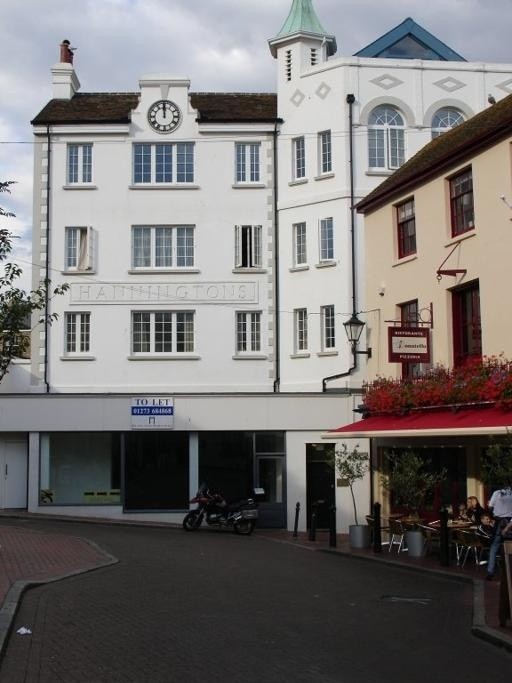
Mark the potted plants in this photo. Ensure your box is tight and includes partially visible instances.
[324,442,371,548]
[376,448,449,557]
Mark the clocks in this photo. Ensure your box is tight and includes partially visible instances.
[147,98,183,134]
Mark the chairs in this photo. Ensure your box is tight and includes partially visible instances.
[387,517,491,568]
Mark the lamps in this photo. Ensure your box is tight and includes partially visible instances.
[343,311,372,358]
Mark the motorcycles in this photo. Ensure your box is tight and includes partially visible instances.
[182,480,260,535]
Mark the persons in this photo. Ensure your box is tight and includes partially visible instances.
[443,476,512,580]
